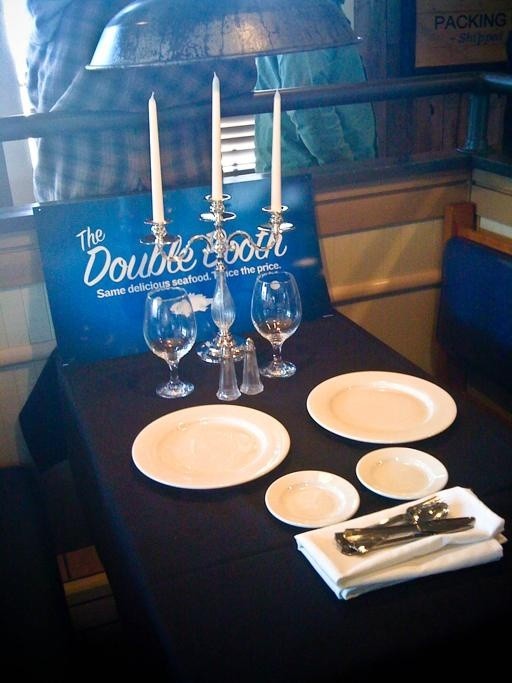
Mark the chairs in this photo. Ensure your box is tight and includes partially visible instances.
[423,193,511,436]
[18,174,335,542]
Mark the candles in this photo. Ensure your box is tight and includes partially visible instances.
[147,88,165,222]
[209,68,223,200]
[271,86,282,210]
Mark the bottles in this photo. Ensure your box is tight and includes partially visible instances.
[242,340,267,395]
[216,344,242,404]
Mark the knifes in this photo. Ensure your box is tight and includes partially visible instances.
[335,495,477,555]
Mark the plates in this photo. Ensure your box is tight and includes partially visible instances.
[132,404,292,489]
[305,370,458,445]
[356,445,447,505]
[264,469,360,528]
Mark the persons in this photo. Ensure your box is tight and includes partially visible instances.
[23,0,258,203]
[254,0,378,173]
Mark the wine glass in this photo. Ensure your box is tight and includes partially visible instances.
[252,271,304,378]
[144,285,197,397]
